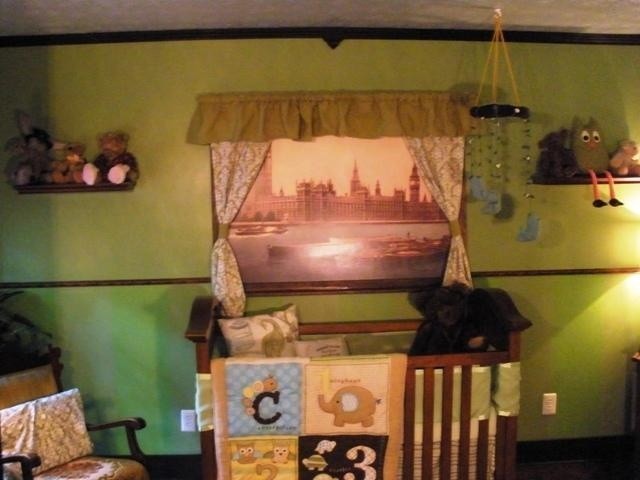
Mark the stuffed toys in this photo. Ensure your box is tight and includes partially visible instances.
[536,116,640,207]
[1,110,139,190]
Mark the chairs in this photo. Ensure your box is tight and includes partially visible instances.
[0,363,147,480]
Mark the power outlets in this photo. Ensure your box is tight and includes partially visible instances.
[542,393,557,416]
[181,410,196,433]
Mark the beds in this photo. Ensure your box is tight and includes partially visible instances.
[184,289,526,478]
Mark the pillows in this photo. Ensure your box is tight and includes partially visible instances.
[295,338,352,360]
[2,388,93,479]
[218,305,297,357]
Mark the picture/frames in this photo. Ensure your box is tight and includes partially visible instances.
[194,89,466,291]
